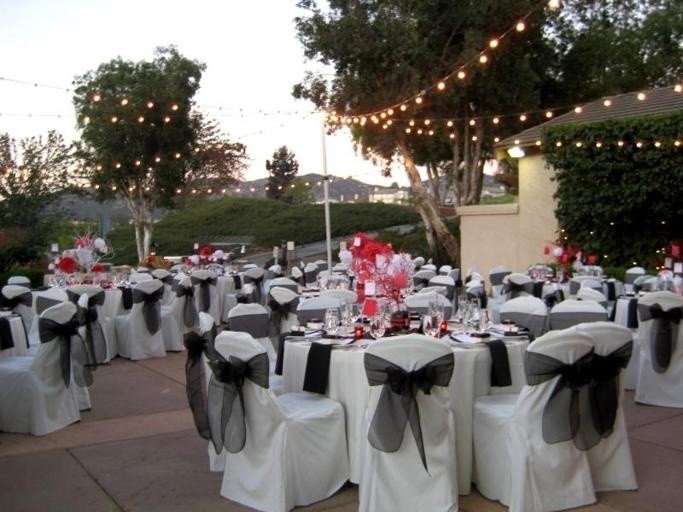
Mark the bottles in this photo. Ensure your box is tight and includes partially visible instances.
[353,324,363,337]
[408,316,421,328]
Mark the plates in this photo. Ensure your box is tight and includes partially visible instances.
[449,329,502,344]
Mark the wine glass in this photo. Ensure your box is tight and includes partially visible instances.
[345,299,364,338]
[457,286,468,321]
[428,301,445,338]
[339,298,348,333]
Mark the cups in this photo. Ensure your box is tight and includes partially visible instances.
[422,314,439,338]
[290,325,305,340]
[368,316,387,339]
[324,307,339,335]
[306,317,322,330]
[409,310,420,316]
[500,320,515,331]
[470,299,481,320]
